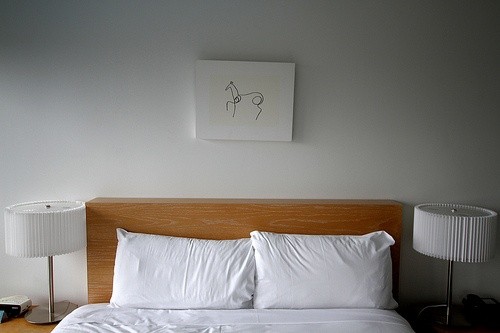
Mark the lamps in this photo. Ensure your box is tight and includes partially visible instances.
[413,202,498,326]
[5,200,86,324]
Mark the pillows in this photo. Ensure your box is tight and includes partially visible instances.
[250,230,398,309]
[107,228,256,309]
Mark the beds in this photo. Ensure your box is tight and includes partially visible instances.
[50,197,416,333]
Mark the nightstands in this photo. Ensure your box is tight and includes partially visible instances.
[401,301,500,333]
[0,306,59,333]
